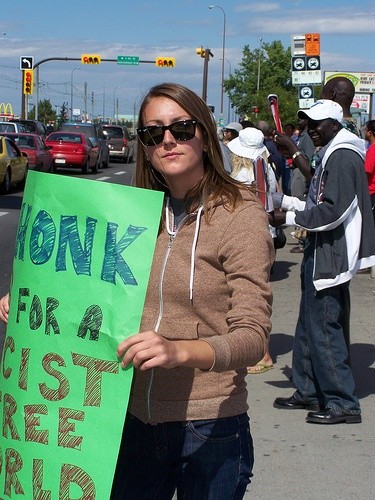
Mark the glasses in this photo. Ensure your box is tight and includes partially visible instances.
[135,119,201,147]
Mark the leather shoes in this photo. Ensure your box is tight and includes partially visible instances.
[272,395,321,412]
[306,408,362,424]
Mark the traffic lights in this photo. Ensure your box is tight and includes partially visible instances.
[24,69,33,95]
[196,45,205,58]
[156,57,176,67]
[252,106,259,113]
[81,54,101,65]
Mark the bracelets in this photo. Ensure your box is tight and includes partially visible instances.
[292,150,303,159]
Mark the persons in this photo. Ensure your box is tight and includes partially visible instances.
[268,98,375,425]
[1,82,276,500]
[221,78,374,251]
[226,127,278,374]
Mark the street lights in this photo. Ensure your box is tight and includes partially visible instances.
[208,4,226,127]
[71,67,81,122]
[219,58,232,124]
[114,86,121,118]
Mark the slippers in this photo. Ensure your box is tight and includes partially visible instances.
[247,363,275,374]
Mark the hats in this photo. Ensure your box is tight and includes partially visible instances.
[224,122,244,134]
[297,98,344,126]
[227,127,270,161]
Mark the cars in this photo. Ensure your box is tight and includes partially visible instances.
[0,136,29,194]
[43,131,100,174]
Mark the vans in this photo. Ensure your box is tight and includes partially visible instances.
[58,121,111,169]
[0,119,55,145]
[102,124,136,163]
